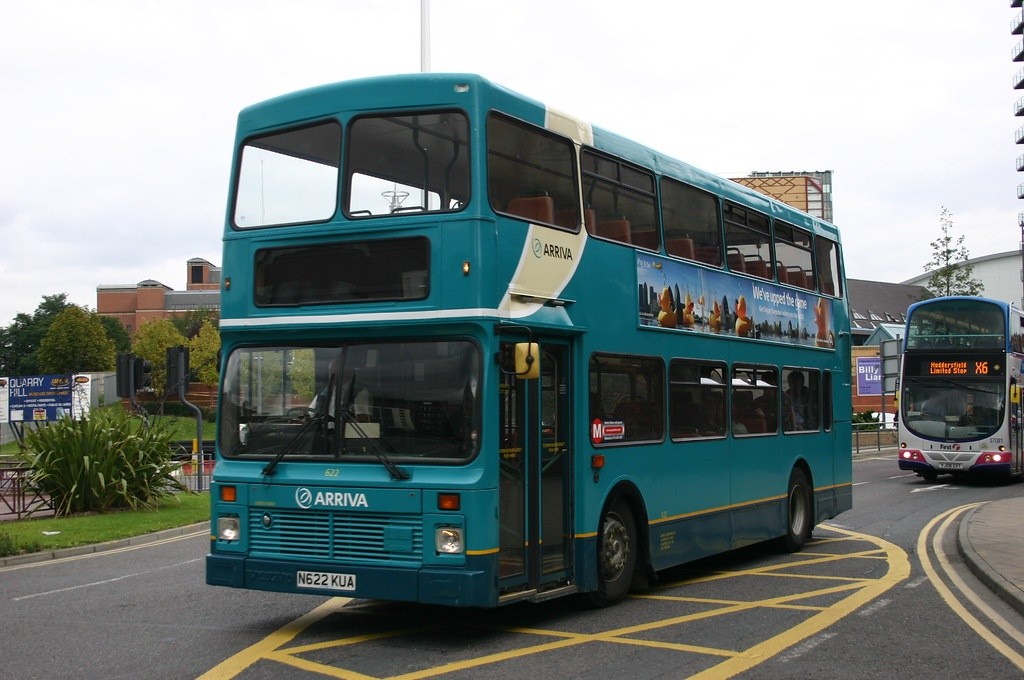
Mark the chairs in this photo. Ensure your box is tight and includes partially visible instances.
[554,203,596,235]
[694,246,719,266]
[736,416,767,434]
[611,396,645,425]
[804,270,826,293]
[631,231,659,251]
[727,248,746,273]
[786,266,807,289]
[744,255,768,279]
[666,231,695,260]
[596,214,632,243]
[765,261,788,284]
[506,189,554,225]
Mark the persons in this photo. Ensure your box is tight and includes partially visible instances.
[308,358,375,424]
[920,393,947,417]
[697,369,816,437]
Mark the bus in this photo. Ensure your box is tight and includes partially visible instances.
[203,73,853,611]
[897,296,1024,484]
